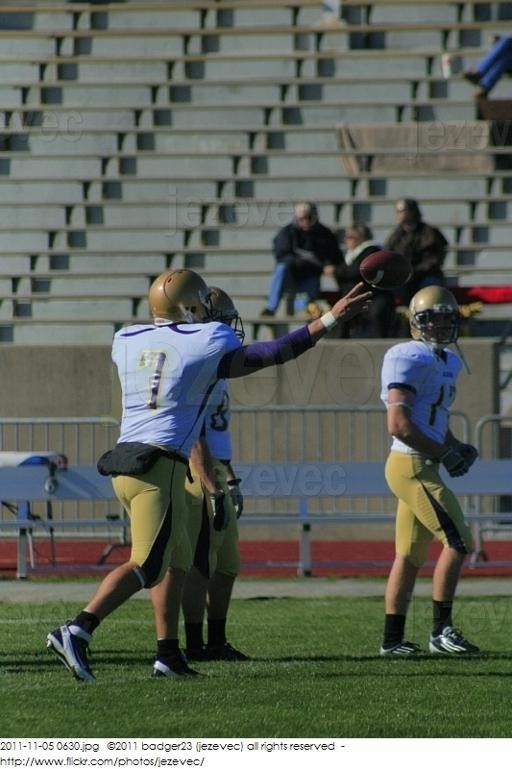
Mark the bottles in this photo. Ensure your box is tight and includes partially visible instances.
[294,293,308,318]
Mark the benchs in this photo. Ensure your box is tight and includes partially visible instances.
[0,0,512,342]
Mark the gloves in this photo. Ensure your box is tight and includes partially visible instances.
[227,475,243,520]
[437,439,477,478]
[206,489,229,531]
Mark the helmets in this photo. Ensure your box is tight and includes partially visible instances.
[149,269,245,345]
[409,285,459,344]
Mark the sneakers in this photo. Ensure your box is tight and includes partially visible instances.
[153,651,203,678]
[429,625,479,653]
[381,641,427,655]
[185,642,250,661]
[44,621,96,680]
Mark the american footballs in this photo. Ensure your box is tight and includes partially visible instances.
[359,250,413,291]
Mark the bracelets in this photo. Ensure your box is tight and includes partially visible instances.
[320,311,337,333]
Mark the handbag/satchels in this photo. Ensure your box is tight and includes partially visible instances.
[97,441,162,473]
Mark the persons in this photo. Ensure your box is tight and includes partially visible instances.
[319,222,396,339]
[180,283,245,663]
[381,285,480,656]
[462,35,512,98]
[382,197,449,293]
[260,200,346,320]
[45,267,374,684]
